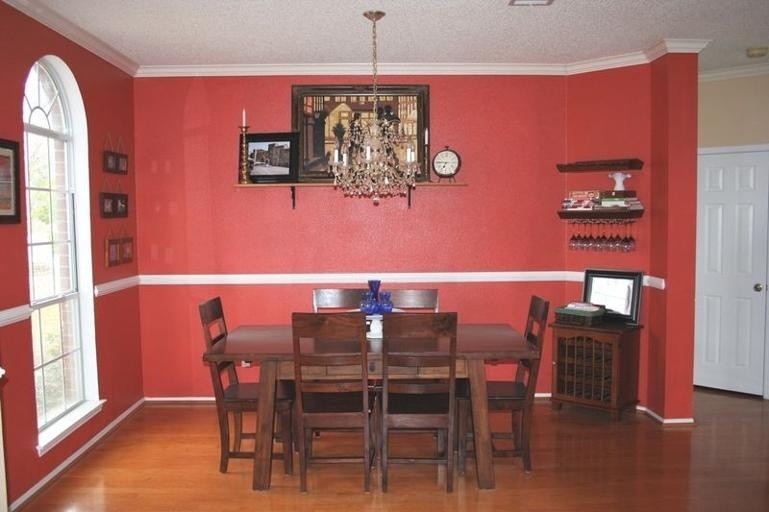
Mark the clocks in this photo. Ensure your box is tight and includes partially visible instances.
[432,146,462,183]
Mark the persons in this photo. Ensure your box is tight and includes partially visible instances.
[383,105,402,129]
[376,107,384,128]
[584,191,599,204]
[119,199,127,213]
[347,112,364,171]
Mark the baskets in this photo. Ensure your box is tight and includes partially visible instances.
[555,305,606,326]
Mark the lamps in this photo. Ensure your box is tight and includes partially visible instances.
[332,10,429,206]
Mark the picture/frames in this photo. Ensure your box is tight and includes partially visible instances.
[100,192,127,217]
[103,151,128,175]
[105,236,134,266]
[291,84,430,183]
[0,139,20,224]
[240,133,298,183]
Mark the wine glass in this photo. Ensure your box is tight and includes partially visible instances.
[569,220,638,251]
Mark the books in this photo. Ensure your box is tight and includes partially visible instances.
[599,190,645,207]
[592,207,645,210]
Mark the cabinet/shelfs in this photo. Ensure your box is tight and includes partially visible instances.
[557,158,644,220]
[548,321,644,421]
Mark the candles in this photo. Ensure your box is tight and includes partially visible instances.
[424,126,429,145]
[242,108,246,126]
